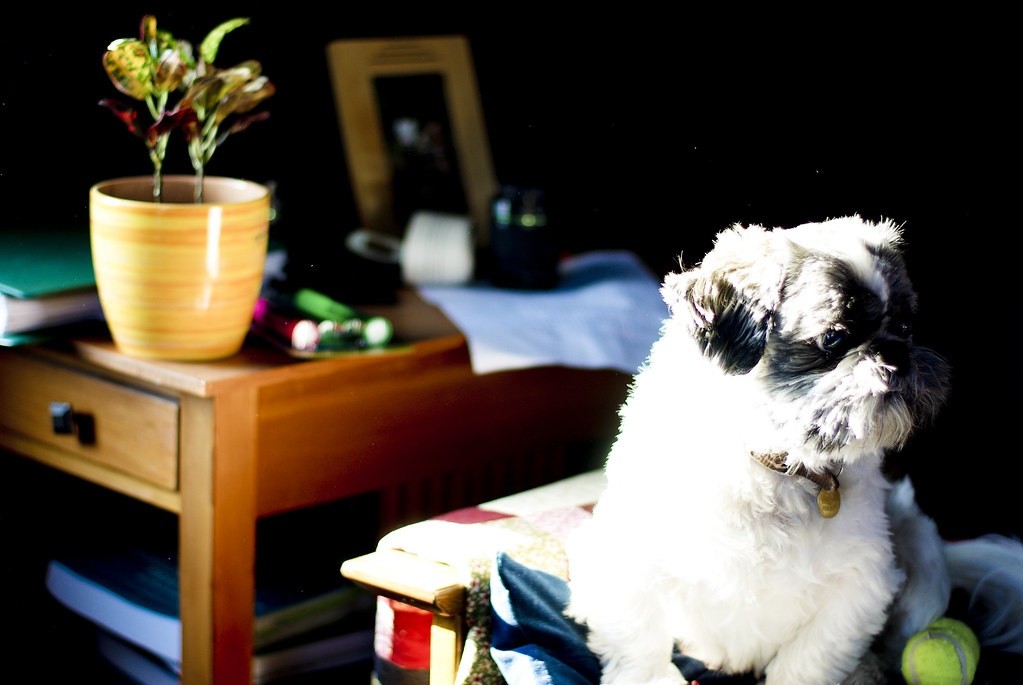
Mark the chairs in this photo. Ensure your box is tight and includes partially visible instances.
[336,467,1023,685]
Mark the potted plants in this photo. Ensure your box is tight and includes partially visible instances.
[89,12,278,365]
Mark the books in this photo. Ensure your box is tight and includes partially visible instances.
[0,230,104,347]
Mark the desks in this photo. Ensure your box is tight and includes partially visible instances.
[0,322,559,685]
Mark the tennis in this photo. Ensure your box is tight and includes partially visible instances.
[901,618,980,685]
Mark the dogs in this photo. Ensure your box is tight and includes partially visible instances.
[560,213,950,685]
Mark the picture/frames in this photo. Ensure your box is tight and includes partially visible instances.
[329,40,498,228]
